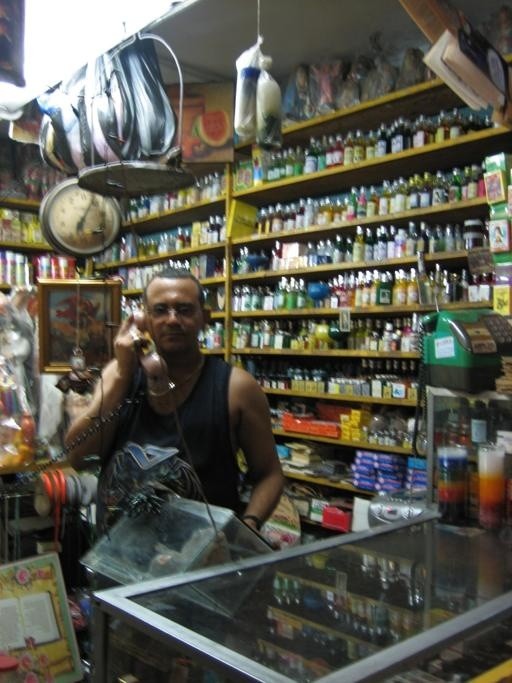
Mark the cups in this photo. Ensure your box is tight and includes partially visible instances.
[434,441,508,533]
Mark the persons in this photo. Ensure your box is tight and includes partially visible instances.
[64,266,285,537]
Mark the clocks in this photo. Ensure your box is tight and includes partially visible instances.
[38,176,126,258]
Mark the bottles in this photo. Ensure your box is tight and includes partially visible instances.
[93,154,225,351]
[230,106,509,399]
[257,554,471,683]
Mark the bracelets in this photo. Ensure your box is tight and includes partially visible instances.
[241,514,264,530]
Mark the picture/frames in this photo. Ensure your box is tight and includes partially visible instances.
[32,276,123,378]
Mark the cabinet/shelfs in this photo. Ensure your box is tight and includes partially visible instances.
[223,53,512,504]
[2,482,68,562]
[260,536,460,681]
[82,512,510,683]
[0,195,87,301]
[119,161,228,359]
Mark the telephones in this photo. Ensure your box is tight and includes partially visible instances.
[124,311,154,357]
[418,305,510,392]
